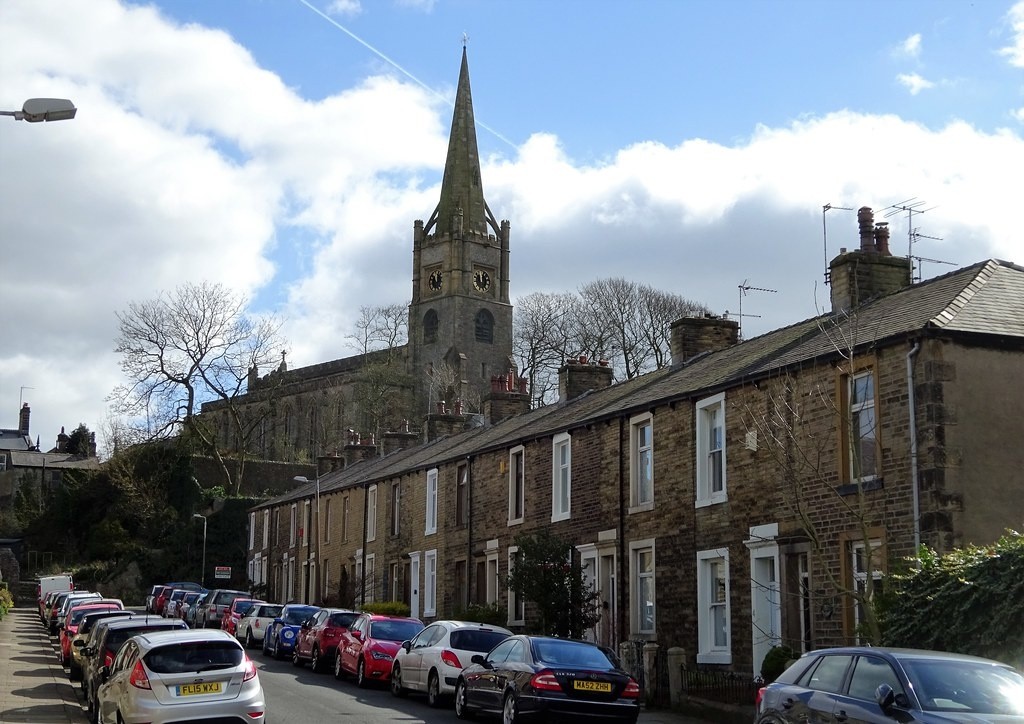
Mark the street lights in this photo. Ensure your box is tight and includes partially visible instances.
[194,514,206,590]
[294,476,322,608]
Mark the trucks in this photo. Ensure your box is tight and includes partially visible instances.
[36,571,73,600]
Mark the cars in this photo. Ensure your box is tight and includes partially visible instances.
[334,612,425,689]
[146,582,284,650]
[754,647,1024,724]
[454,635,641,724]
[39,591,267,724]
[390,621,516,708]
[292,608,362,673]
[263,603,321,661]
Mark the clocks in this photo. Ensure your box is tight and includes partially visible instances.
[428,269,444,293]
[473,270,491,293]
